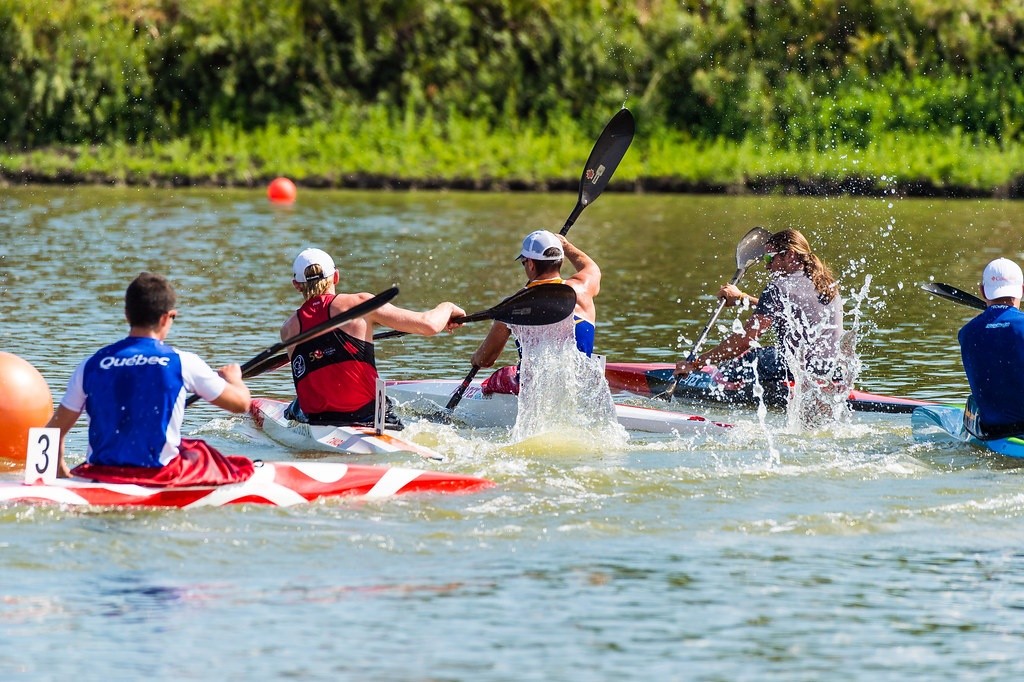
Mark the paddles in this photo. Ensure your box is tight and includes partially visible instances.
[237,282,580,379]
[180,286,406,413]
[437,107,641,427]
[917,275,991,311]
[650,227,778,405]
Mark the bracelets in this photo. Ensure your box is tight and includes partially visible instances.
[738,293,747,306]
[692,357,703,371]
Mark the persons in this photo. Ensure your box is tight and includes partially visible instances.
[44,272,252,478]
[471,229,601,396]
[957,256,1024,440]
[279,248,467,431]
[673,228,843,383]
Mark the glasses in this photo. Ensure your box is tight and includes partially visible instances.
[169,314,175,320]
[521,257,528,265]
[763,250,789,264]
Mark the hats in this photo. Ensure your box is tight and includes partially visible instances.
[515,229,564,261]
[292,247,336,283]
[982,256,1024,300]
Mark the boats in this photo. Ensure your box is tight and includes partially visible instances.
[605,360,935,439]
[382,376,735,452]
[910,403,1024,480]
[1,457,495,508]
[249,395,474,460]
